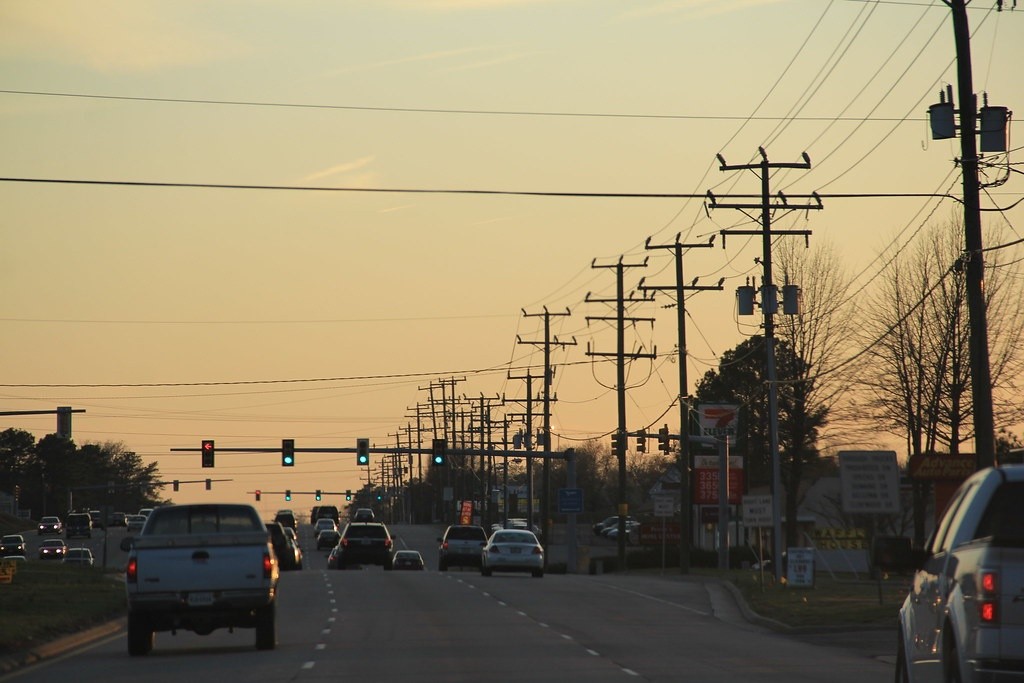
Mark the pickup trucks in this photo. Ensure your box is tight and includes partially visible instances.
[120,503,279,655]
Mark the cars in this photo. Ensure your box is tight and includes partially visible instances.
[0,535,27,560]
[479,529,544,577]
[354,508,374,521]
[38,539,67,560]
[63,548,95,565]
[314,518,341,569]
[89,509,154,532]
[265,509,303,570]
[601,521,641,541]
[491,519,542,539]
[392,549,424,570]
[37,516,62,535]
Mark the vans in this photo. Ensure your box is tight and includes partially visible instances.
[65,513,93,539]
[309,506,341,525]
[897,464,1024,683]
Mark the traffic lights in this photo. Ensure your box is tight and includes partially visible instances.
[376,490,382,501]
[658,424,669,450]
[286,490,291,501]
[202,440,214,467]
[206,479,211,490]
[433,438,445,466]
[282,439,294,466]
[637,429,646,453]
[256,490,261,501]
[316,490,321,501]
[611,433,619,459]
[346,490,351,501]
[357,439,369,465]
[174,480,178,491]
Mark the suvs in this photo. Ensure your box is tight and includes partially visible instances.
[335,522,396,570]
[436,524,488,571]
[592,515,633,536]
